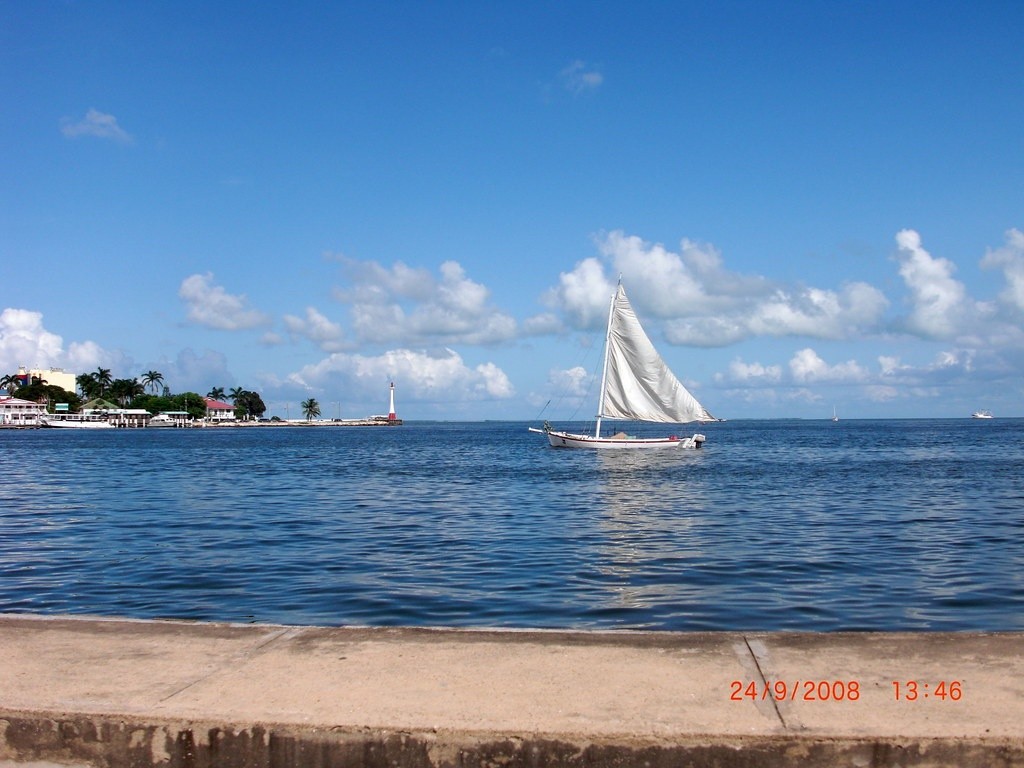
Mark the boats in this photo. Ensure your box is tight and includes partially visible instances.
[40,418,116,428]
[147,414,175,427]
[971,406,995,419]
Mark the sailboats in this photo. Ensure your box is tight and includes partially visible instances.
[832,406,839,422]
[527,271,727,450]
